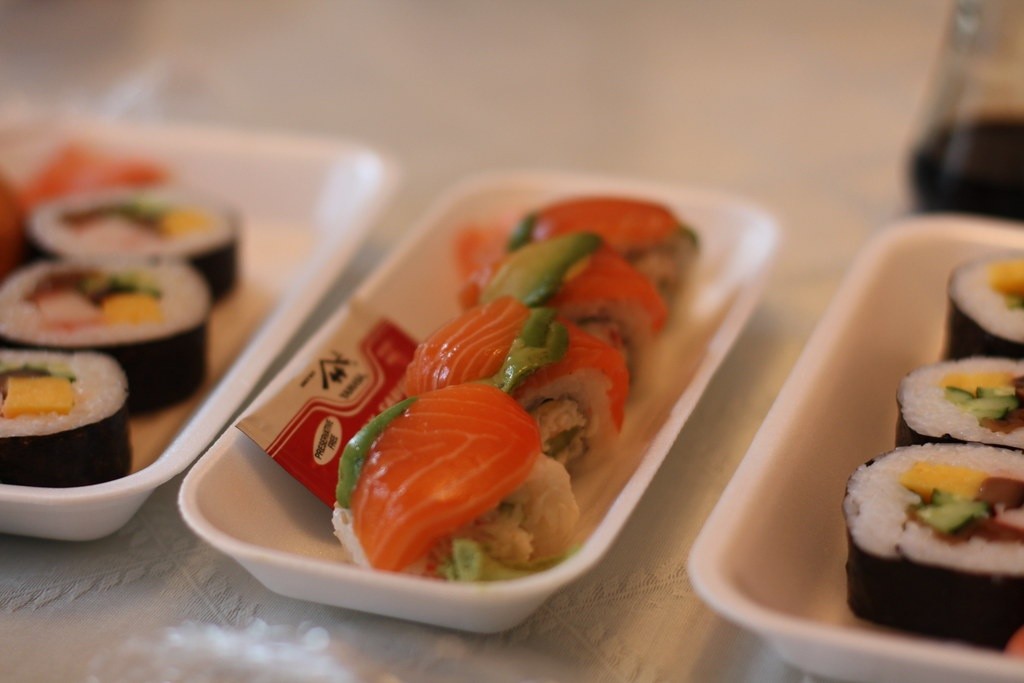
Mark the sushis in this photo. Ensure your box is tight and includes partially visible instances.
[328,198,701,577]
[843,250,1023,654]
[0,182,237,488]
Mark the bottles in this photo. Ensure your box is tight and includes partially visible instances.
[902,0,1024,223]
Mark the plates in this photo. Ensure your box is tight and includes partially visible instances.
[685,214,1024,683]
[176,170,781,633]
[0,97,397,544]
